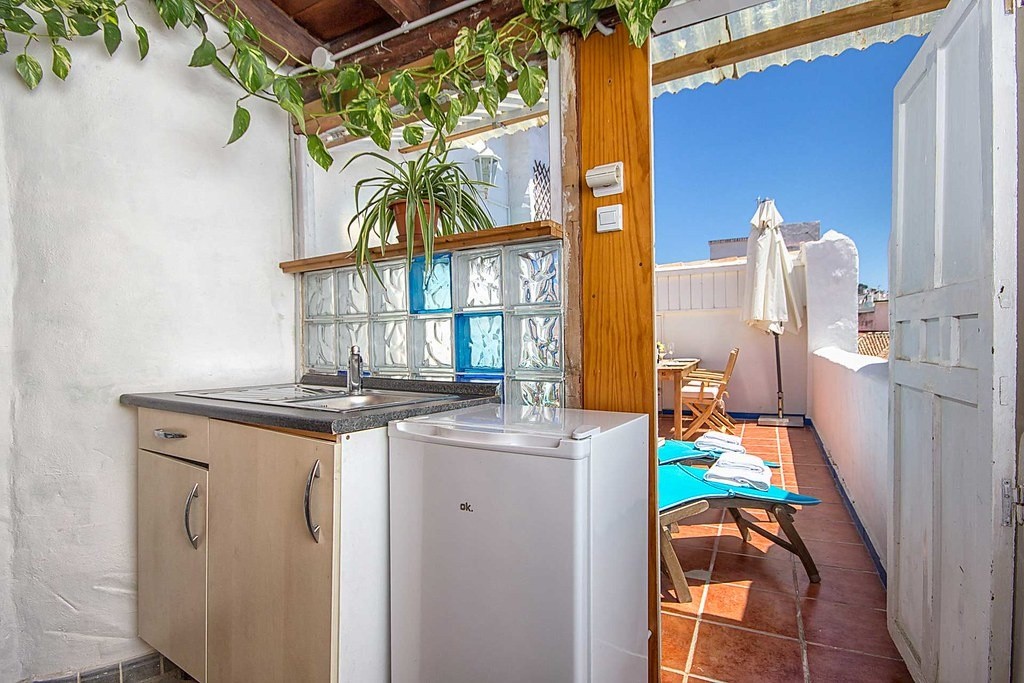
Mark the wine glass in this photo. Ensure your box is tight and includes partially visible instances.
[668,342,675,362]
[658,350,667,364]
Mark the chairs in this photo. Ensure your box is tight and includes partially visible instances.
[657,441,823,604]
[682,347,739,441]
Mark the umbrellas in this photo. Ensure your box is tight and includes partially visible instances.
[739,198,803,418]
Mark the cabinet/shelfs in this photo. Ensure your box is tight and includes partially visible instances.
[121,407,502,682]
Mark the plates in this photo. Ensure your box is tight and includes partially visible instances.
[663,362,687,366]
[673,359,697,362]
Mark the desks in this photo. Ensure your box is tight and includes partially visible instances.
[657,358,700,441]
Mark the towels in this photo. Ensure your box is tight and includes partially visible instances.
[694,430,746,454]
[702,452,771,492]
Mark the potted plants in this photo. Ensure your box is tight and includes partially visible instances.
[339,127,500,293]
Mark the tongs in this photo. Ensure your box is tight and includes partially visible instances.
[294,385,345,396]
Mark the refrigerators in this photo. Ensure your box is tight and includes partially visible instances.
[388,403,649,683]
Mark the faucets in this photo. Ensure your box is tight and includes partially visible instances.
[346,345,364,395]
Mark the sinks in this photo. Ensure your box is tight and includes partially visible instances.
[286,391,460,415]
